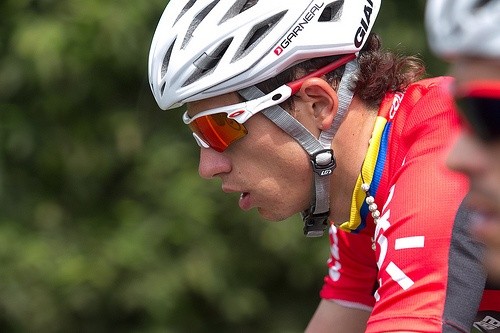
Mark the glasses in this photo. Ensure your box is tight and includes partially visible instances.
[183,52,360,153]
[444,81,500,143]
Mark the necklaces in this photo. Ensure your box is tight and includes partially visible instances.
[360,131,381,250]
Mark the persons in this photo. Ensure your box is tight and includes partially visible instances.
[147,0,475,333]
[424,0,500,333]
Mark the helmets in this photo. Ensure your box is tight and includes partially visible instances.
[149,0,381,111]
[425,0,500,60]
